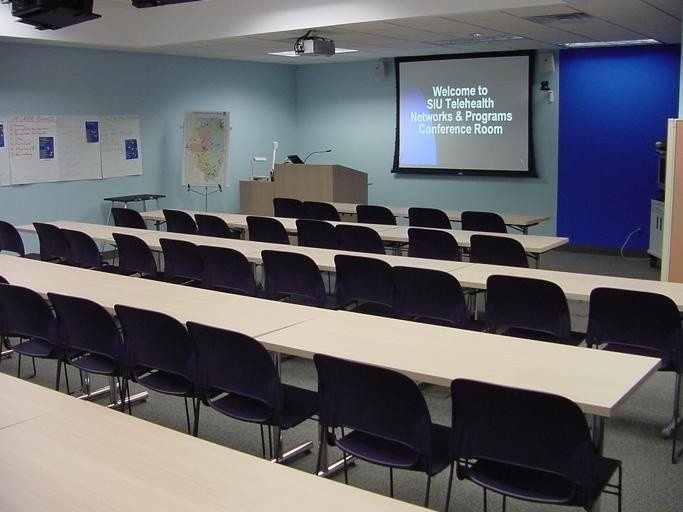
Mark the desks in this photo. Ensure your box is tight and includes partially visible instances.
[1,246,662,478]
[1,371,430,508]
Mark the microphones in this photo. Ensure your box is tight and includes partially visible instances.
[304,149,332,163]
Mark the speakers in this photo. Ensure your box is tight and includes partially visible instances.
[374,62,386,80]
[538,53,555,73]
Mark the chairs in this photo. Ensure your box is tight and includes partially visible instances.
[184,318,352,485]
[1,280,83,393]
[42,290,122,414]
[1,189,586,349]
[442,375,624,508]
[310,351,490,508]
[112,299,206,437]
[587,281,680,468]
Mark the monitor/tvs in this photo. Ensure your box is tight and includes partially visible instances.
[657,154,666,190]
[286,154,305,164]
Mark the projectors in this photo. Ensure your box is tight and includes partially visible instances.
[294,37,335,57]
